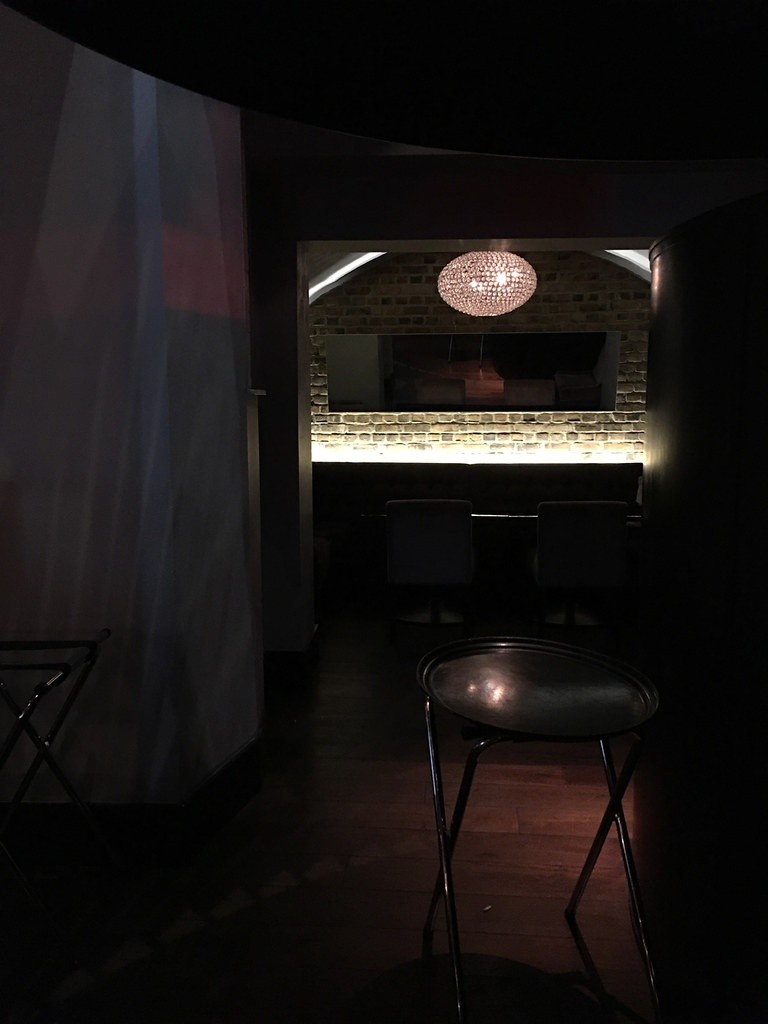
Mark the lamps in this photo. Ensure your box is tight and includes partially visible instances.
[438,251,537,318]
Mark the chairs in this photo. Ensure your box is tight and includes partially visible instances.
[363,496,477,627]
[529,500,628,626]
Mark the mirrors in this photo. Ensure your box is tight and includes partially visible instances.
[324,334,618,413]
